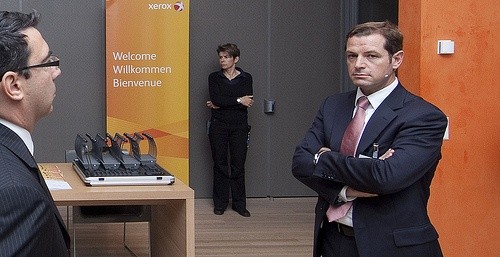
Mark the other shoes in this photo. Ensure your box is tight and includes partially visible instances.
[214,207,224,215]
[232,207,250,217]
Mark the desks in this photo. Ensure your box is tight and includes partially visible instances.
[37,163,195,257]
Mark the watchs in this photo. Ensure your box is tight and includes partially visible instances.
[236,97,241,104]
[313,149,326,166]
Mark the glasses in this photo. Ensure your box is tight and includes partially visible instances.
[12,55,60,72]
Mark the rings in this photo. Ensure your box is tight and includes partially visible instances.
[250,104,252,106]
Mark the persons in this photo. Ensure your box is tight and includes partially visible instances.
[0,10,72,257]
[291,21,448,257]
[207,43,255,218]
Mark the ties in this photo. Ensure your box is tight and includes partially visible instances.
[326,97,370,222]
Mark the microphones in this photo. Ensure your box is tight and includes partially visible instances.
[384,69,396,78]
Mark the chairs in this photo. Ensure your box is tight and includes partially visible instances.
[64,150,150,257]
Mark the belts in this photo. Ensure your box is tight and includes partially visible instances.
[324,219,354,236]
